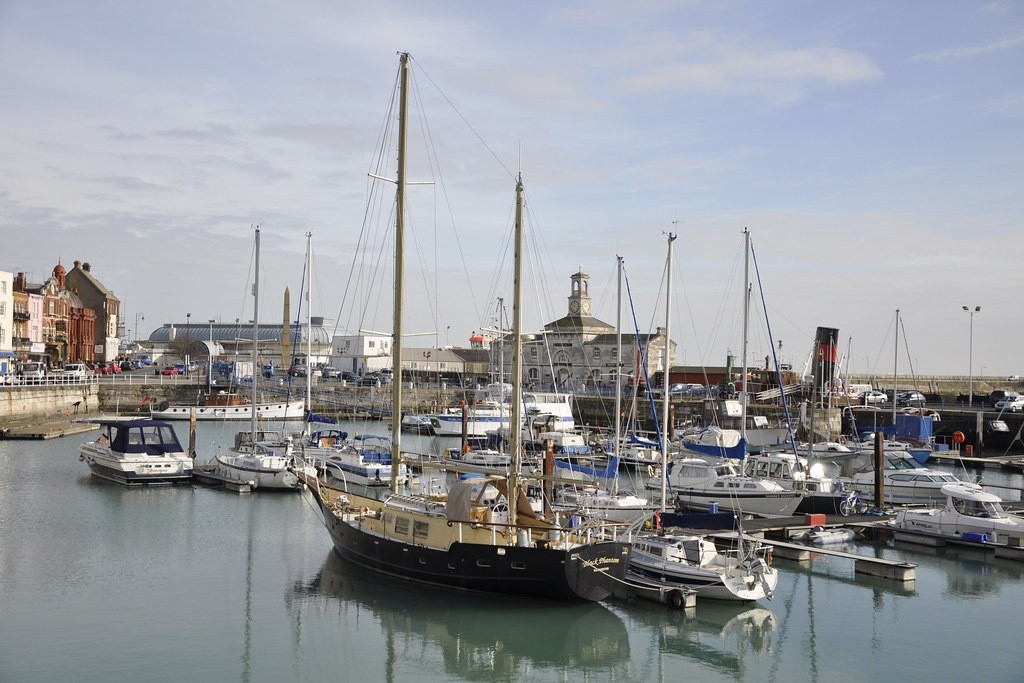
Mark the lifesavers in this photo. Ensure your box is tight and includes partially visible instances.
[664,588,687,611]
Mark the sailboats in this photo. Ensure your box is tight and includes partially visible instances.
[572,219,780,602]
[603,283,983,514]
[643,225,806,517]
[215,224,318,492]
[460,296,539,477]
[552,253,662,530]
[285,50,634,604]
[280,231,348,473]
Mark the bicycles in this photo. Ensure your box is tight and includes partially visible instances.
[840,489,869,517]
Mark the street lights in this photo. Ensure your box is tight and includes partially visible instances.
[962,306,981,407]
[136,313,145,344]
[186,313,191,380]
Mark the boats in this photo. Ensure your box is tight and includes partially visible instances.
[76,400,194,487]
[326,434,407,487]
[148,390,306,422]
[789,525,856,545]
[483,405,589,455]
[424,382,576,436]
[884,483,1024,562]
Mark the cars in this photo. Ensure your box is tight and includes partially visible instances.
[21,355,151,384]
[858,391,926,408]
[668,383,719,396]
[989,390,1024,413]
[262,363,393,387]
[161,361,196,375]
